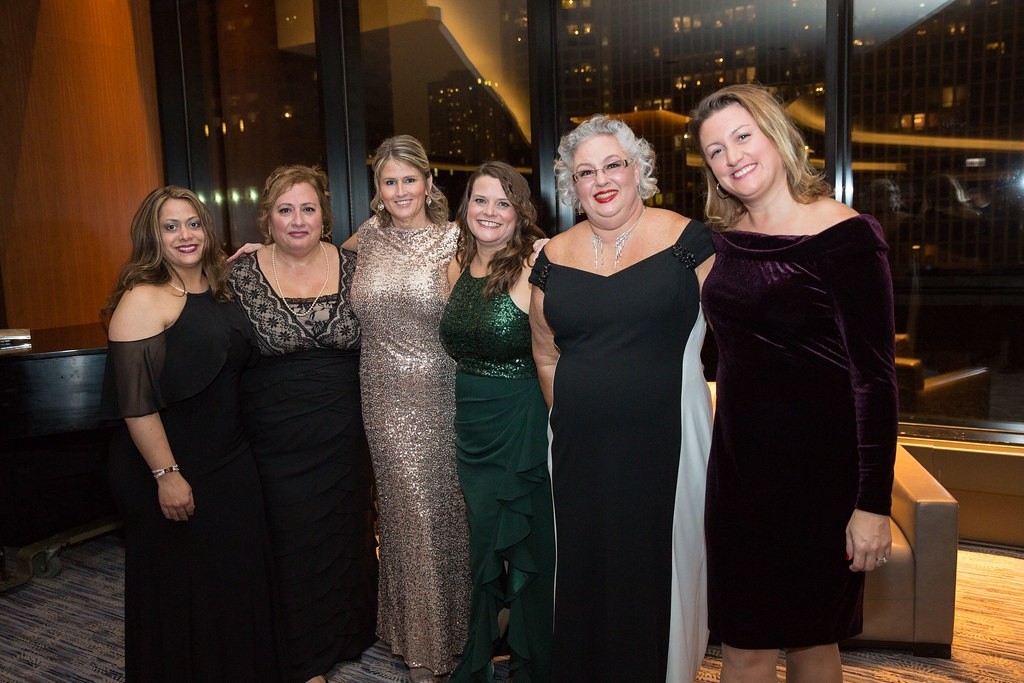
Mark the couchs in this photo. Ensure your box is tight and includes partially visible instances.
[707,382,960,659]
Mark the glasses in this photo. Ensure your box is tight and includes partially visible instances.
[572,158,634,183]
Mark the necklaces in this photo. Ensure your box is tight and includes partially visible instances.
[272,240,329,316]
[592,206,647,271]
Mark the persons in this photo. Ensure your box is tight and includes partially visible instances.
[98,185,285,683]
[436,161,556,683]
[690,86,898,683]
[226,165,380,683]
[527,118,715,683]
[226,134,551,683]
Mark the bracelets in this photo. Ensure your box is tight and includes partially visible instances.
[151,465,179,479]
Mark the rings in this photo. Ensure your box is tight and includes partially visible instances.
[877,555,887,563]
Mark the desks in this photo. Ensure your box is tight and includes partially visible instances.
[0,322,144,572]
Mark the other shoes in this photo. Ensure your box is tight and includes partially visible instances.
[409,667,441,683]
